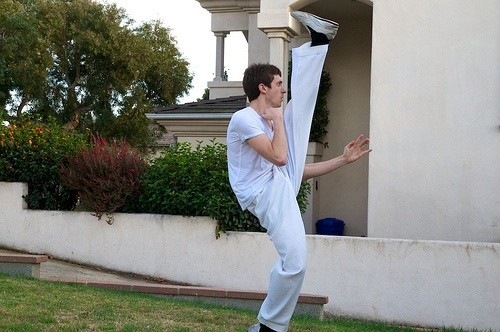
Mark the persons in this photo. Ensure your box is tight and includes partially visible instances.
[225,10,373,332]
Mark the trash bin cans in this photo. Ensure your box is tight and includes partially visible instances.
[315,217,345,235]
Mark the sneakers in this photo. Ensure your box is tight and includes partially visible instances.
[291,10,339,41]
